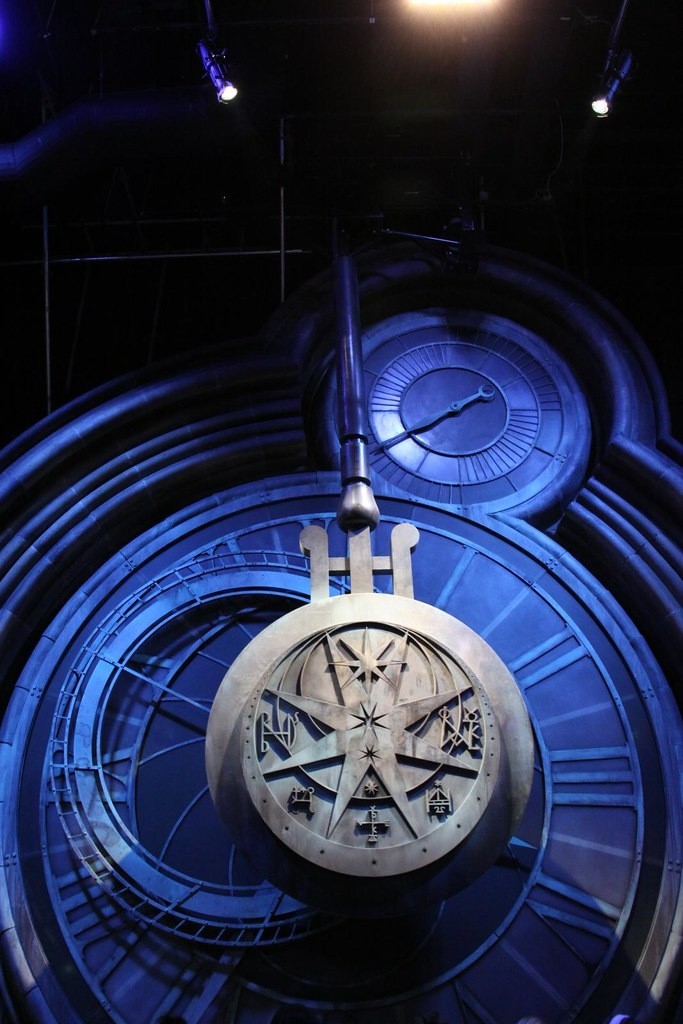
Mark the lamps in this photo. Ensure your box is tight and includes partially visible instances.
[196,0,238,105]
[589,0,633,119]
